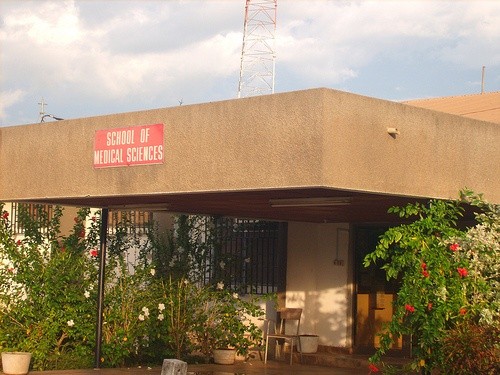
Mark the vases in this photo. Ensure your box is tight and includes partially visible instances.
[296,335,319,354]
[2,352,32,375]
[214,349,235,364]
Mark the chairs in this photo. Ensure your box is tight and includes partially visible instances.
[265,308,302,368]
